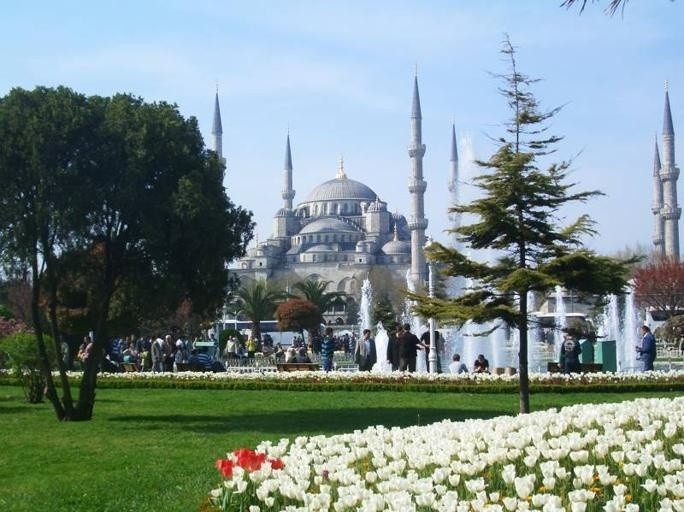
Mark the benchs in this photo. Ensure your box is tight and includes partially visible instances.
[276,363,320,371]
[547,363,603,374]
[123,362,138,372]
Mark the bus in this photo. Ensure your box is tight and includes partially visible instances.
[537,311,597,335]
[212,319,326,346]
[644,304,684,330]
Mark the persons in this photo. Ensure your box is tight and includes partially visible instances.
[387,323,429,373]
[354,329,377,372]
[473,355,489,373]
[560,334,582,374]
[637,326,656,371]
[449,354,468,375]
[322,328,337,371]
[333,332,359,358]
[59,334,312,372]
[420,323,443,373]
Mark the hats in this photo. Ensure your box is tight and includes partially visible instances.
[404,324,411,330]
